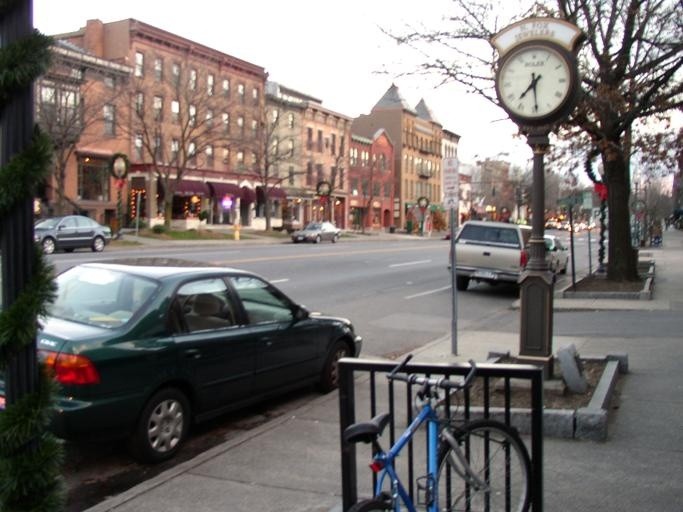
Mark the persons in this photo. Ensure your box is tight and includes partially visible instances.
[654,225,662,247]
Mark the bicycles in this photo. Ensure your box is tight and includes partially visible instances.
[343,353,531,512]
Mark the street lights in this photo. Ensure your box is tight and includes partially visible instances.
[417,197,429,236]
[110,152,129,241]
[316,180,331,221]
[631,200,646,246]
[585,147,607,265]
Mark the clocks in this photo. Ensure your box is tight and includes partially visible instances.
[494,39,578,126]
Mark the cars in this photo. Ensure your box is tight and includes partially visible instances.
[448,221,569,290]
[0,263,361,464]
[34,215,112,254]
[291,222,342,243]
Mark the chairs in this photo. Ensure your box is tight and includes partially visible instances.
[61,285,230,332]
